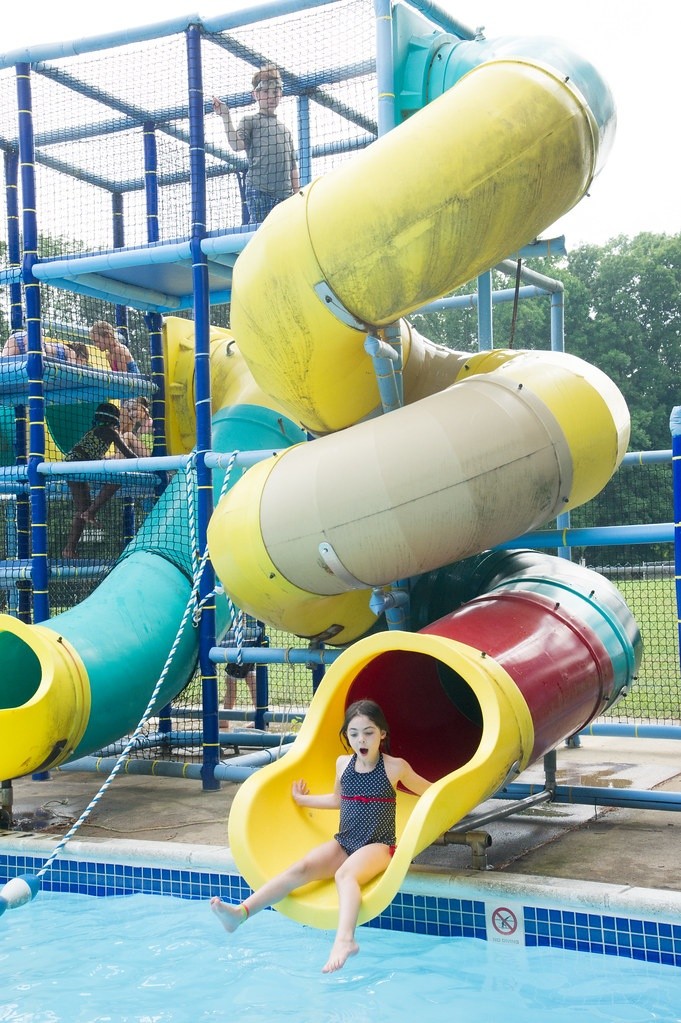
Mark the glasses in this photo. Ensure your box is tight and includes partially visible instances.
[254,79,283,92]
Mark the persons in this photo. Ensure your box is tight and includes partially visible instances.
[209,699,434,974]
[61,403,139,559]
[218,612,257,728]
[212,69,301,224]
[2,330,89,365]
[89,320,154,459]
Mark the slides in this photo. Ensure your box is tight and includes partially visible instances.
[0,318,311,788]
[204,29,648,935]
